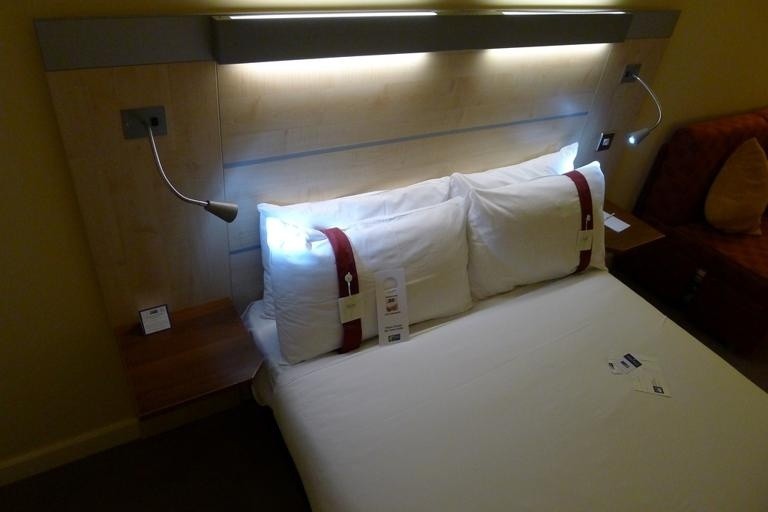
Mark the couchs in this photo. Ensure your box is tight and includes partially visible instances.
[630,107,768,359]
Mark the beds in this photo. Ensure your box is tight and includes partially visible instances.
[242,270,768,511]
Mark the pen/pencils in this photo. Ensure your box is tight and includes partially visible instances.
[604,212,615,221]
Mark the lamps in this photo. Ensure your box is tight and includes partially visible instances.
[212,9,632,64]
[121,106,238,223]
[621,62,663,148]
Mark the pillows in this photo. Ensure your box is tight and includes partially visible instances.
[705,139,768,241]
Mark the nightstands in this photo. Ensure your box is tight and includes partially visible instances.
[602,198,666,258]
[114,298,270,421]
